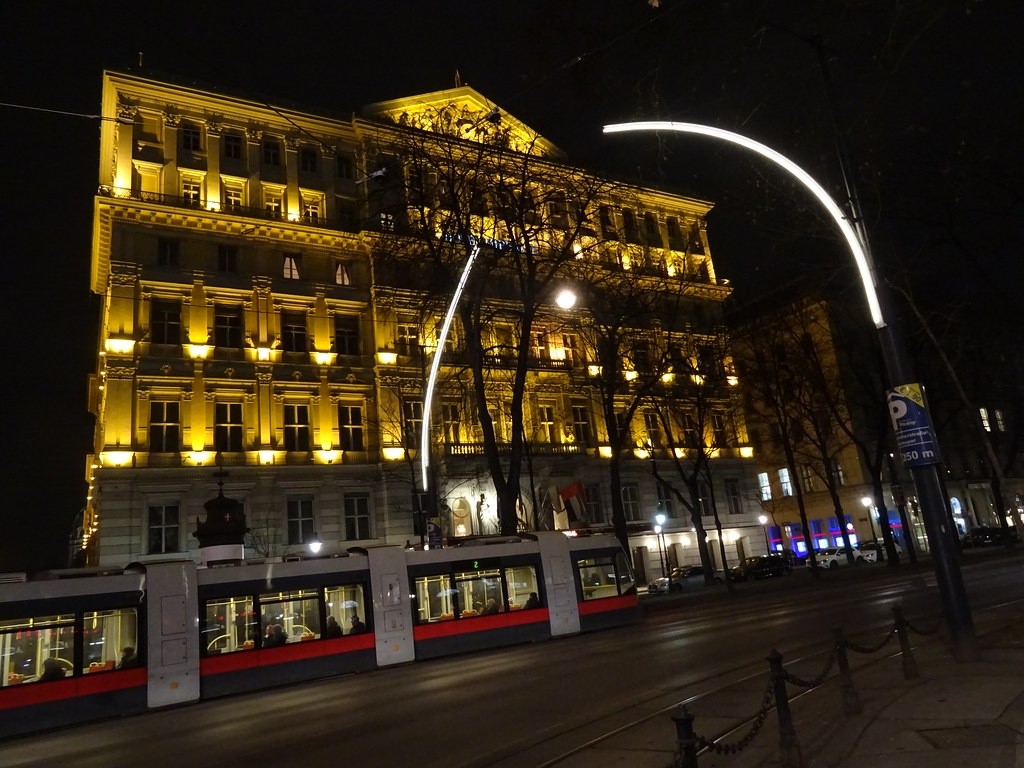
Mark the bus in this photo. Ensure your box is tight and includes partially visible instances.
[0,529,644,745]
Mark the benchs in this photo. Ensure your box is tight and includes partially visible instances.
[87,660,115,672]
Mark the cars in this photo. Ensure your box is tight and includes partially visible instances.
[806,546,867,572]
[727,556,765,581]
[856,541,904,563]
[648,564,727,598]
[744,555,794,581]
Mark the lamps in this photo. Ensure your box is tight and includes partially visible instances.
[309,530,322,553]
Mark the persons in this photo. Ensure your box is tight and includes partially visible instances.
[116,646,137,669]
[472,592,539,616]
[263,613,366,647]
[41,658,65,681]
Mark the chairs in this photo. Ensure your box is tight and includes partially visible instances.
[300,632,315,641]
[242,641,254,649]
[9,674,24,685]
[440,612,454,620]
[501,603,522,611]
[462,610,476,617]
[89,661,105,666]
